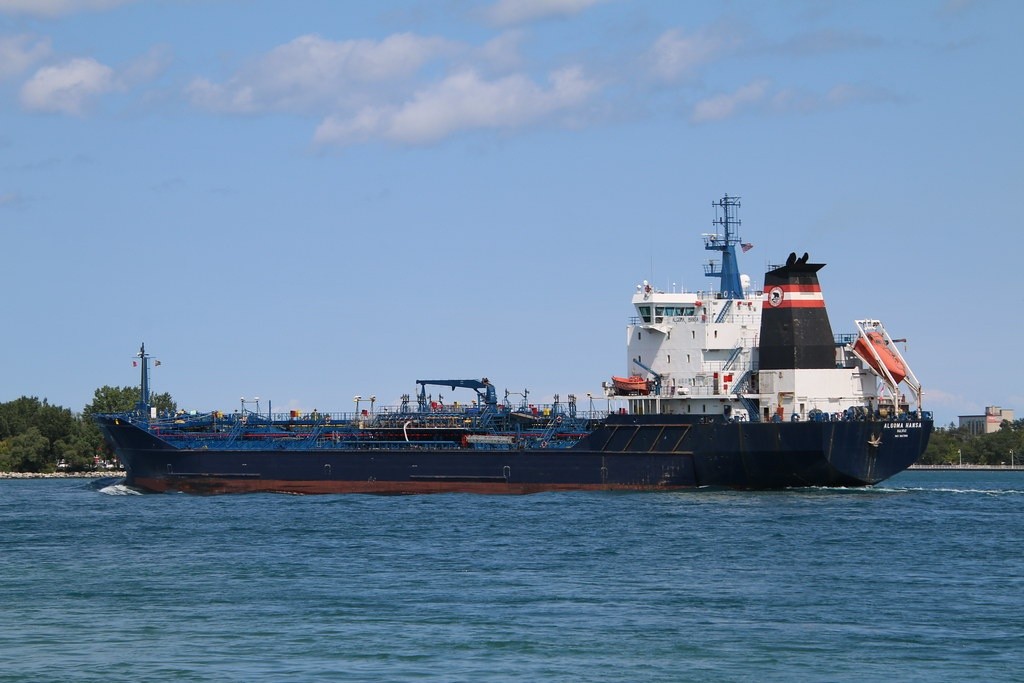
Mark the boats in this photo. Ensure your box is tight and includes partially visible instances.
[89,195,933,493]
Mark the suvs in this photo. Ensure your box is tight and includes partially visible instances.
[935,460,953,465]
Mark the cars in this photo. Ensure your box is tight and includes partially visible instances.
[55,459,127,473]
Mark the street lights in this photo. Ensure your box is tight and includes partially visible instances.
[1010,448,1014,469]
[958,448,962,468]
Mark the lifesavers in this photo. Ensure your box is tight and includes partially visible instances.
[556,416,562,423]
[541,441,547,448]
